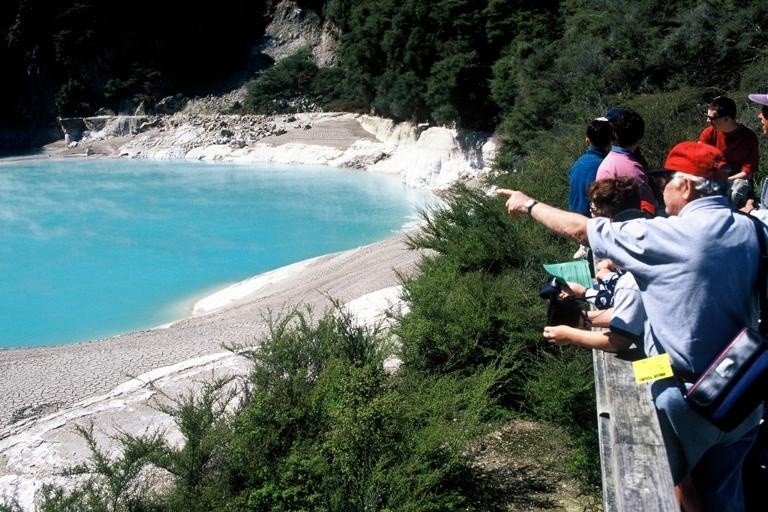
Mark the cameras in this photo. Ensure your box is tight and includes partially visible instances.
[730,178,751,206]
[540,273,568,302]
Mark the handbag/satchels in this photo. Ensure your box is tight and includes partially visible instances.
[540,277,584,325]
[683,325,768,433]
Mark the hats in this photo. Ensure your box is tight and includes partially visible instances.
[647,140,728,181]
[595,108,624,122]
[748,94,768,106]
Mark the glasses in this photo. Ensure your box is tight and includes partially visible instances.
[704,113,723,120]
